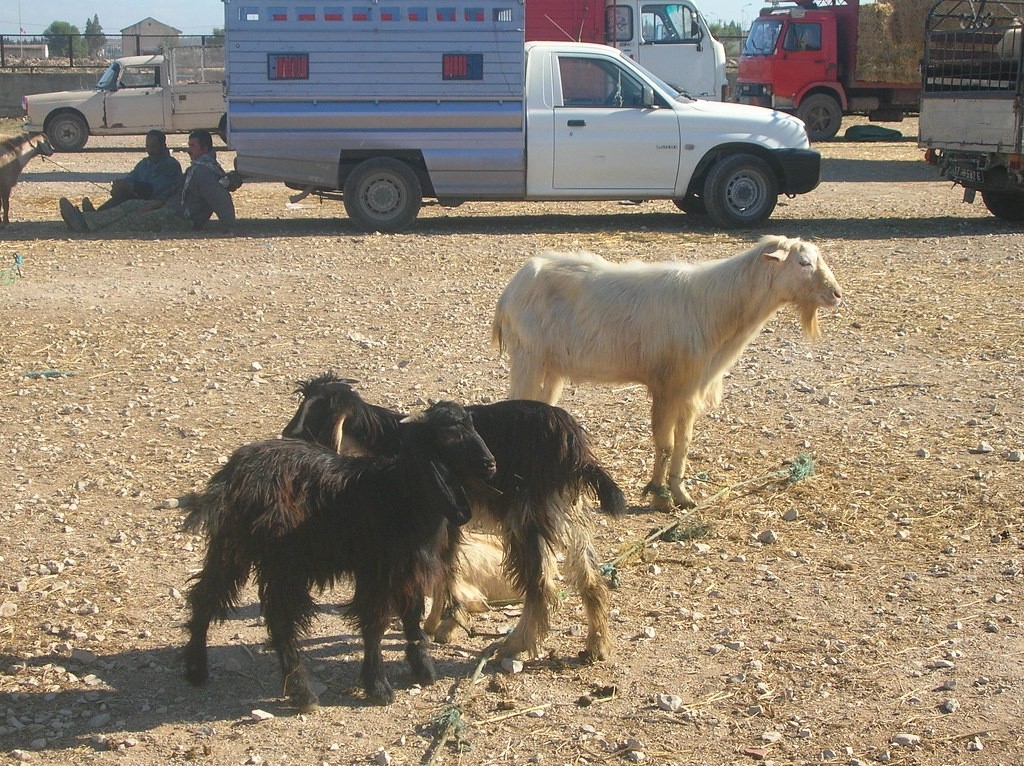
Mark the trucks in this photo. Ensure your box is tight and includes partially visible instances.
[526,2,730,100]
[224,1,821,230]
[736,0,923,140]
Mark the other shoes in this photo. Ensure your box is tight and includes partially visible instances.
[82,197,96,212]
[60,197,87,233]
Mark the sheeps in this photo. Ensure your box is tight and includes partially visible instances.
[177,232,843,714]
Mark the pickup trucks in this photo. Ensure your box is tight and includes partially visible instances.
[20,49,227,154]
[916,1,1024,223]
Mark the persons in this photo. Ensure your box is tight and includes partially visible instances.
[59,130,248,238]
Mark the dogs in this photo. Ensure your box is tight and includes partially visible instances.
[0,131,55,225]
